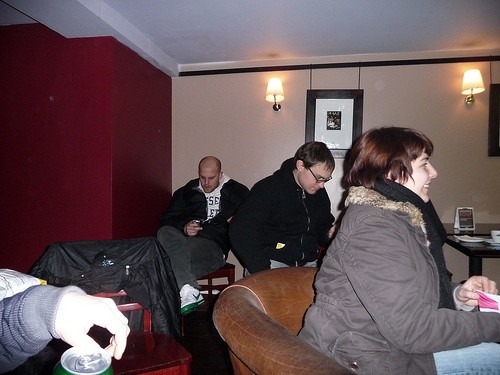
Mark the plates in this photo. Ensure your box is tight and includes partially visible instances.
[484,237,500,248]
[452,234,486,243]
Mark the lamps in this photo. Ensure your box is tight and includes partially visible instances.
[461,69,485,105]
[264,77,284,112]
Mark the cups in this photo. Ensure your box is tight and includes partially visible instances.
[490,230,500,243]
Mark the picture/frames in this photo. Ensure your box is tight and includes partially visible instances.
[305,89,364,160]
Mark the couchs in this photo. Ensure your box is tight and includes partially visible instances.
[214,266,355,375]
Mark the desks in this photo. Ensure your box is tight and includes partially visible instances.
[443,222,500,277]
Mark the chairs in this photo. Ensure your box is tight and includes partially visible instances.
[181,263,236,336]
[91,290,193,375]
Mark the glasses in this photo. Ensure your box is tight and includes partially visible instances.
[303,161,332,183]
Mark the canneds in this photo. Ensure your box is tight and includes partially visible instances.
[54,345,113,375]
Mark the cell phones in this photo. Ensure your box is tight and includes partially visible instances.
[195,220,202,225]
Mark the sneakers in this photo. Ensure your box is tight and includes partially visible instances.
[179,284,205,316]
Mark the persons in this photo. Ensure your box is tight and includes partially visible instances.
[156,156,250,314]
[296,126,500,375]
[228,141,339,278]
[0,284,130,375]
